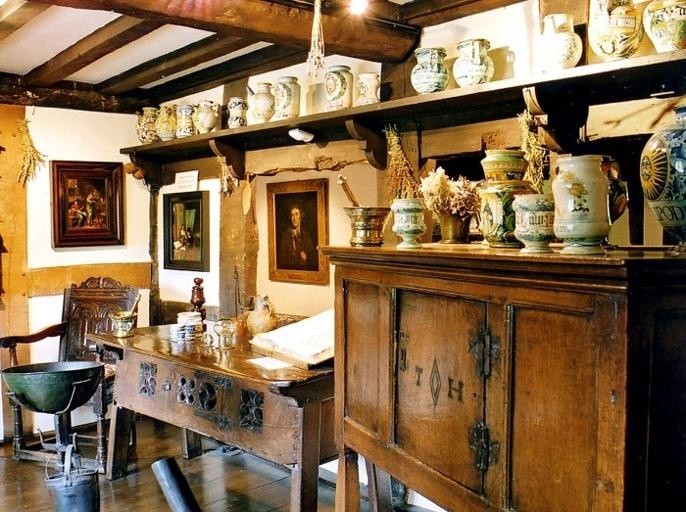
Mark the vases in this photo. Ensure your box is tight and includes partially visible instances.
[641,1,684,52]
[407,45,450,96]
[319,64,354,111]
[310,98,686,258]
[136,98,220,145]
[448,35,496,88]
[273,75,302,119]
[532,12,585,72]
[252,81,276,123]
[352,70,382,107]
[225,95,248,128]
[586,1,644,61]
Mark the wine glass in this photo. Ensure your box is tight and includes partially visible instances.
[601,156,629,250]
[213,322,238,351]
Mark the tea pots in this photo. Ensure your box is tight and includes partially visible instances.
[246,295,279,335]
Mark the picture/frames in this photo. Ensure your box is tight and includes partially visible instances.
[265,179,332,284]
[163,189,205,272]
[47,158,125,249]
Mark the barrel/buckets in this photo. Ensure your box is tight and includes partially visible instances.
[43,444,101,512]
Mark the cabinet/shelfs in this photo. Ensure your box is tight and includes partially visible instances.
[318,239,686,511]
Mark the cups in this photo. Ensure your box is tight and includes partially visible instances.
[108,311,137,338]
[476,149,529,183]
[479,181,537,250]
[551,155,612,257]
[390,197,426,251]
[512,193,555,255]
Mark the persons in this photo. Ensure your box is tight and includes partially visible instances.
[276,202,315,271]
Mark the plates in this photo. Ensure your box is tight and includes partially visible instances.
[177,311,203,332]
[170,323,195,342]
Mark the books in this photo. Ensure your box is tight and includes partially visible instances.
[250,306,336,371]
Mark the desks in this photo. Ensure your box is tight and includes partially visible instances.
[81,317,339,512]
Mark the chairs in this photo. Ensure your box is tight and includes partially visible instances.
[0,275,143,476]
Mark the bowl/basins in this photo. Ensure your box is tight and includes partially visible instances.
[2,361,108,413]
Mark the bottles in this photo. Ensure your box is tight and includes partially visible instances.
[452,38,495,88]
[249,45,448,125]
[639,106,686,256]
[534,0,686,91]
[134,95,245,146]
